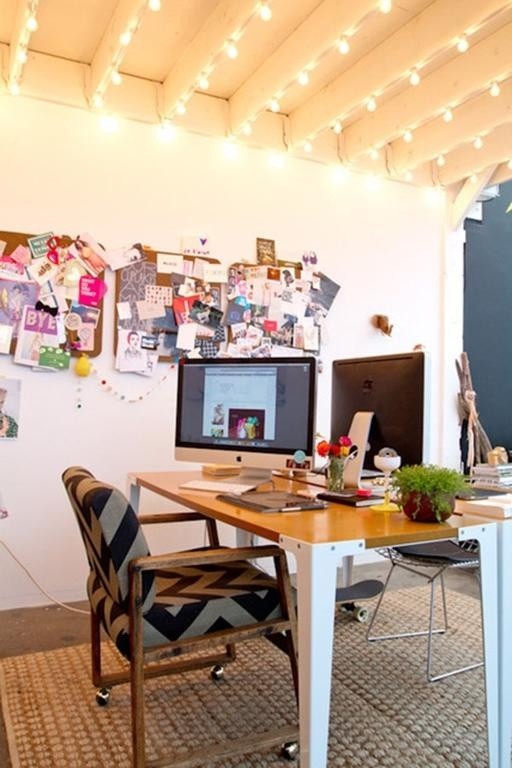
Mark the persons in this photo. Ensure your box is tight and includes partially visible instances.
[123,331,144,361]
[75,239,107,273]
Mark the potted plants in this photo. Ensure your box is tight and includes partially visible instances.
[384,463,476,525]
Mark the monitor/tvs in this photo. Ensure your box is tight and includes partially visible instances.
[326,350,426,491]
[173,354,316,496]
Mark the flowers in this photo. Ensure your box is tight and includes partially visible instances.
[315,435,358,493]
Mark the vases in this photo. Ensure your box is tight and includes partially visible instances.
[326,455,345,493]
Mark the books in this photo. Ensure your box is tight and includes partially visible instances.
[178,478,257,498]
[317,492,384,507]
[13,304,64,372]
[471,461,512,491]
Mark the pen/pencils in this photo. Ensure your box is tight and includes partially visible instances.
[279,505,329,512]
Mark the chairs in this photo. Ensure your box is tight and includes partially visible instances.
[62,466,297,768]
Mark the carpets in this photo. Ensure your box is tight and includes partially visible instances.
[2,582,490,768]
